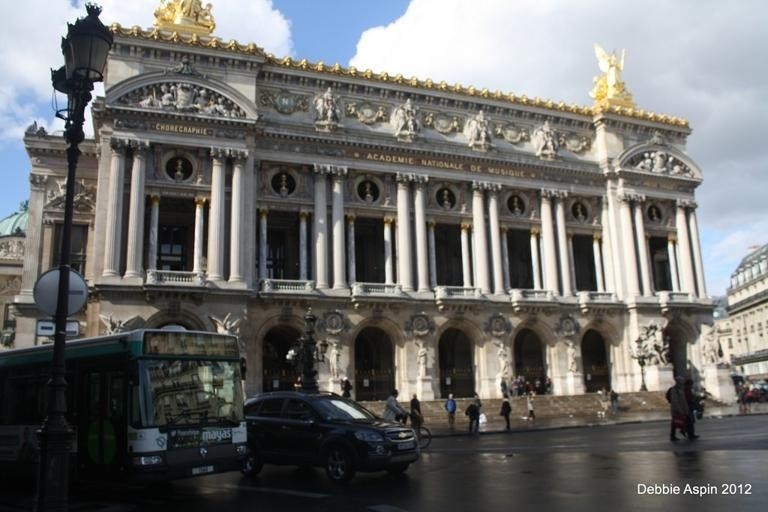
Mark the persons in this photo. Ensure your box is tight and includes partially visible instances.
[330,344,341,377]
[684,378,706,439]
[314,87,341,121]
[390,98,423,132]
[473,394,481,414]
[445,394,456,429]
[294,370,421,437]
[665,377,696,441]
[463,110,494,147]
[567,343,577,372]
[736,376,768,404]
[417,343,427,376]
[532,120,559,155]
[465,400,479,433]
[497,344,509,374]
[500,376,553,431]
[610,390,618,411]
[600,387,608,411]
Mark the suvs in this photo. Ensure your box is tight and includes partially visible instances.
[239,387,420,487]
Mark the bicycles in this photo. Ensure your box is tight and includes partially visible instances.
[402,414,431,450]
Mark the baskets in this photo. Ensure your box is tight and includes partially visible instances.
[412,415,424,424]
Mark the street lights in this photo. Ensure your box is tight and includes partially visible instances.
[34,2,112,509]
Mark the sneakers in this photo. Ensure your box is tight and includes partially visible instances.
[670,430,699,441]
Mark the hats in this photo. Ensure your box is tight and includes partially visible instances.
[505,394,508,398]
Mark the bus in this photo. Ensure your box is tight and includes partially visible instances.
[0,329,252,500]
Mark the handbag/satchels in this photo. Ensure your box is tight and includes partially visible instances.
[500,411,504,416]
[666,388,672,404]
[672,416,688,427]
